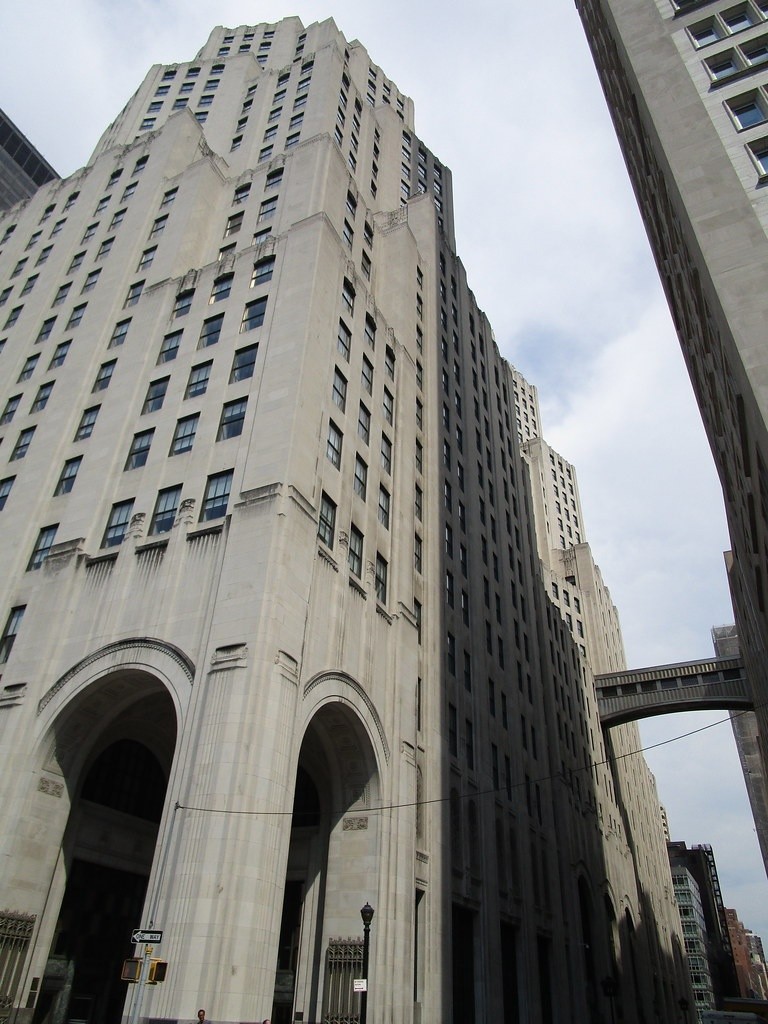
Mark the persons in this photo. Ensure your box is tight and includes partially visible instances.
[197,1010,211,1024]
[263,1020,271,1024]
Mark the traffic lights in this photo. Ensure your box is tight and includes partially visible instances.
[148,961,169,982]
[121,958,143,983]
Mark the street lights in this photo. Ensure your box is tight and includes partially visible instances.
[359,902,375,1024]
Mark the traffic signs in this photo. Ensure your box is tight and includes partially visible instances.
[131,929,164,945]
